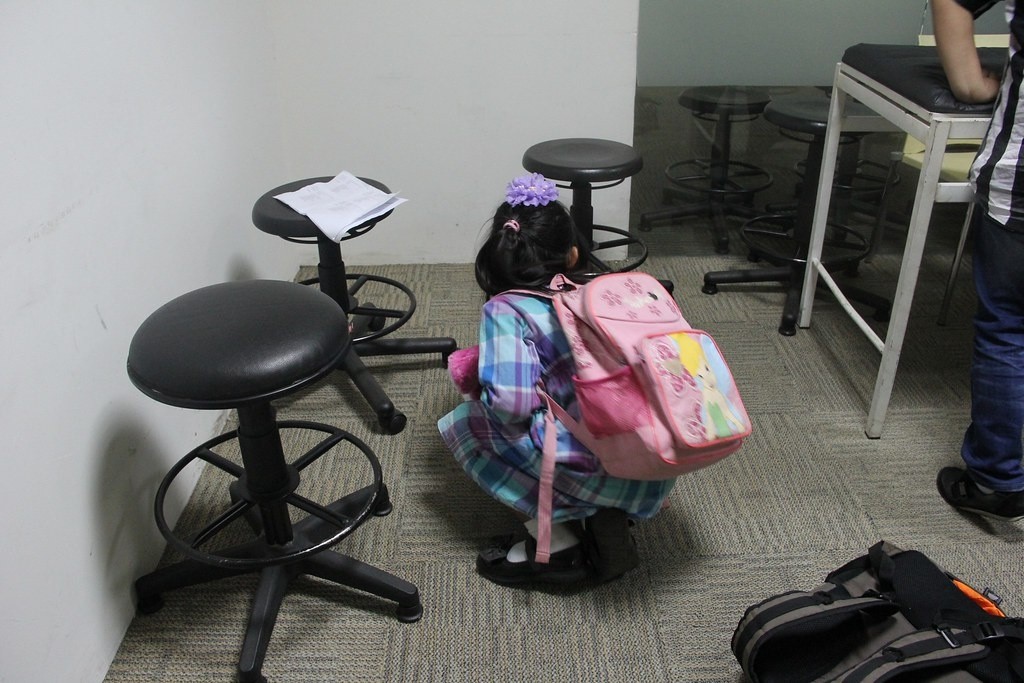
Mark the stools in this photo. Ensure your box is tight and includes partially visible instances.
[639,86,904,337]
[521,138,677,295]
[253,176,460,437]
[127,278,424,683]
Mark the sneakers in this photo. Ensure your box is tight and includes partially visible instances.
[937,466,1024,522]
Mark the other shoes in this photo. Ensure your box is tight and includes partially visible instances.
[476,533,593,589]
[583,505,640,584]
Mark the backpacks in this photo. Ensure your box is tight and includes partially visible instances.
[730,541,1024,683]
[494,271,752,480]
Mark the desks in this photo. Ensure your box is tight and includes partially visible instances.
[796,41,1008,437]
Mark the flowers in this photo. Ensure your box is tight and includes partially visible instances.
[506,173,559,208]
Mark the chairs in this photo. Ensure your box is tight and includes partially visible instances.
[902,34,1010,327]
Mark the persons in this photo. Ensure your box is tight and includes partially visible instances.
[438,172,678,584]
[931,0,1024,522]
